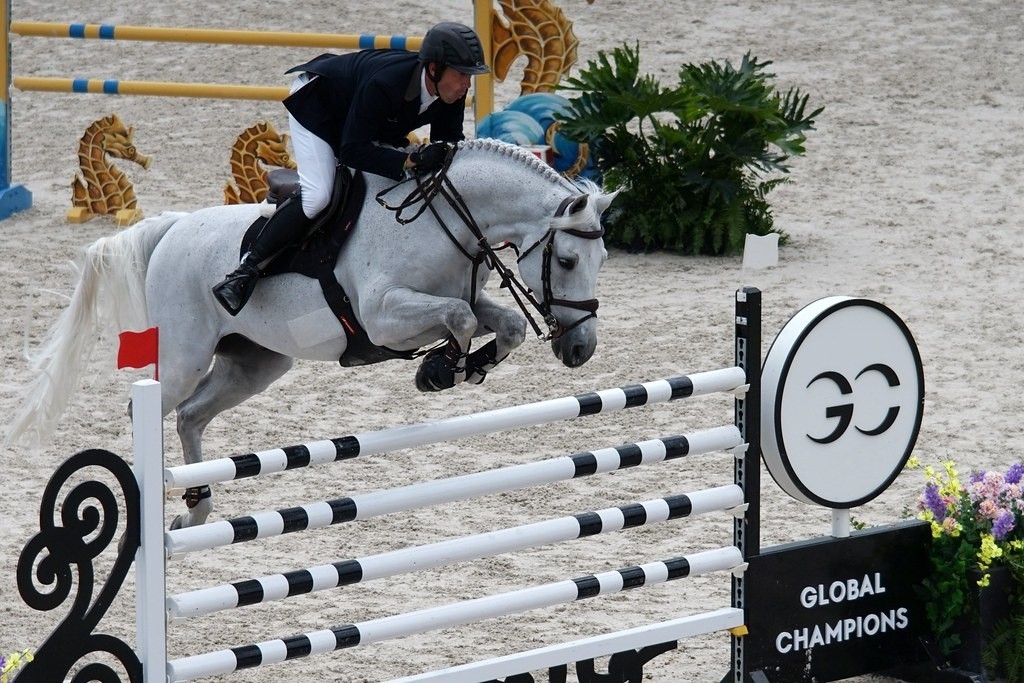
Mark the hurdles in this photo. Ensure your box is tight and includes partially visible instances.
[0,0,590,225]
[13,233,941,683]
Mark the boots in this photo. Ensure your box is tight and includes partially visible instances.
[219,186,311,312]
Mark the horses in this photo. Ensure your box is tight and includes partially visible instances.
[4,137,623,535]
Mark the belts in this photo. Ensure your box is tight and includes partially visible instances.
[307,71,316,81]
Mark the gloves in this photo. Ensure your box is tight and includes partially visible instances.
[410,143,451,175]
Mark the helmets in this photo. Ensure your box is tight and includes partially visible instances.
[418,23,490,74]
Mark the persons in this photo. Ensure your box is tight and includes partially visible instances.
[220,23,490,308]
